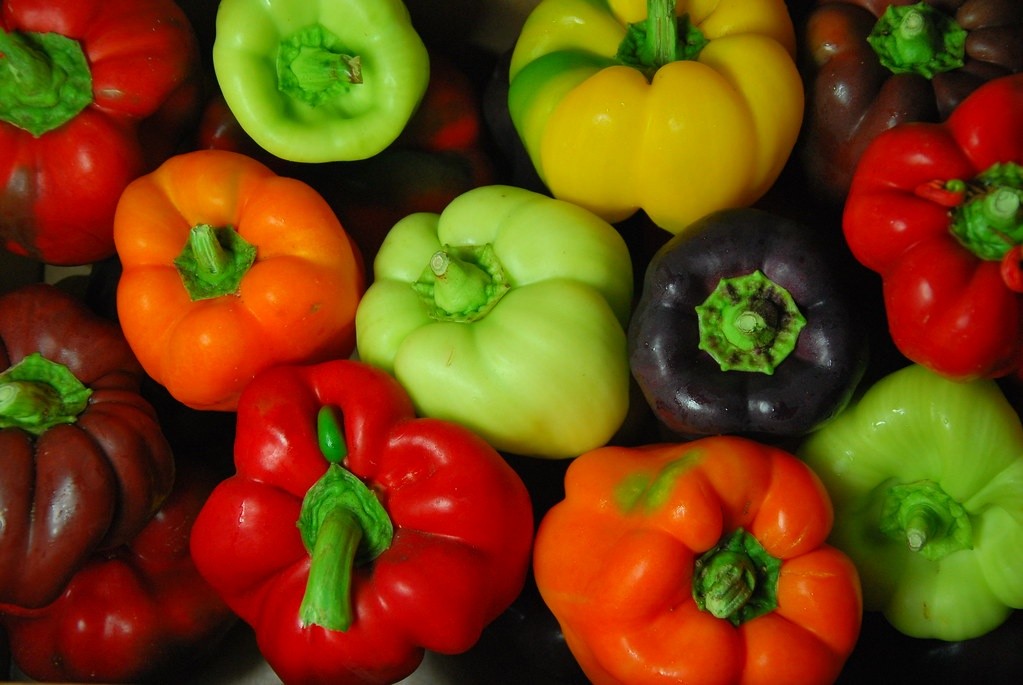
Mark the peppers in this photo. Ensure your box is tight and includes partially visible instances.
[0,0,1023,685]
